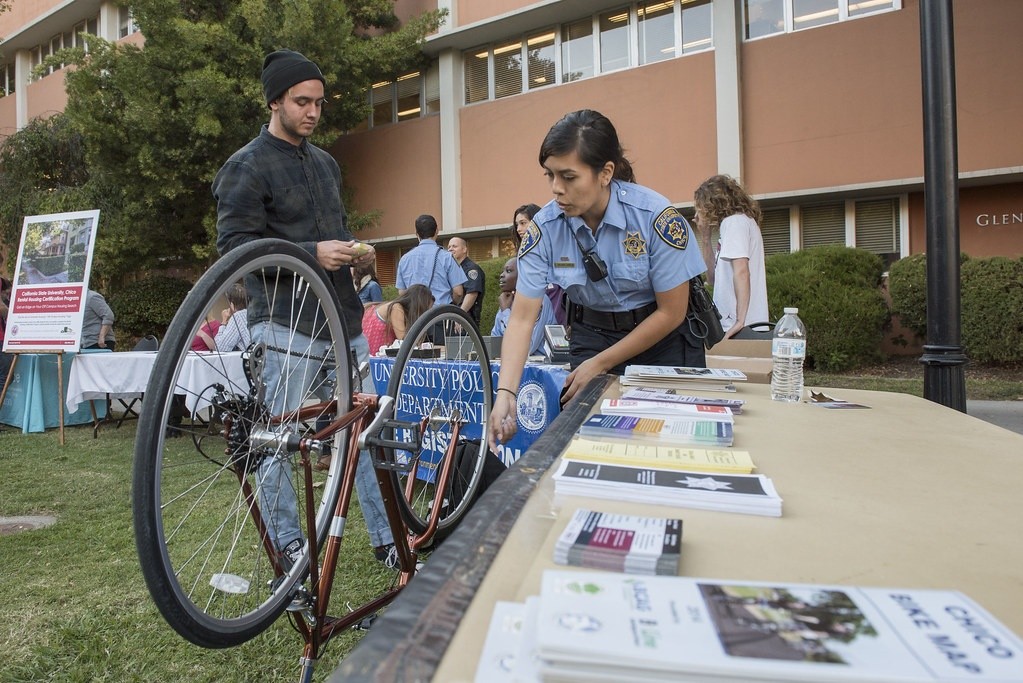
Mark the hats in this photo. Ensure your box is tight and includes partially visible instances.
[261,50,326,110]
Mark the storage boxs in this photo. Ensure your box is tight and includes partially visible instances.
[445,336,503,361]
[385,348,441,358]
[706,338,773,384]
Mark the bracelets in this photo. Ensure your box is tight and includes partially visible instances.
[496,388,516,397]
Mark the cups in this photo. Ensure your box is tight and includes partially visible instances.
[422,343,434,349]
[379,345,387,355]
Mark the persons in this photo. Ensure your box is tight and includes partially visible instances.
[691,175,770,340]
[211,48,425,581]
[487,109,707,455]
[0,252,14,397]
[490,202,571,357]
[165,283,256,439]
[314,215,485,471]
[80,287,116,407]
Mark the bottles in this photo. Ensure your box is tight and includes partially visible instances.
[771,307,807,402]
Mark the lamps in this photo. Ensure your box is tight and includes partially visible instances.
[608,3,670,24]
[475,32,556,60]
[390,71,420,84]
[666,0,698,7]
[362,80,391,92]
[661,37,710,55]
[778,0,893,28]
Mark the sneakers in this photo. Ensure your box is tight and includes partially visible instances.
[374,544,425,576]
[282,539,322,581]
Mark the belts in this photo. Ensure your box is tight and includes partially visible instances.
[575,301,658,332]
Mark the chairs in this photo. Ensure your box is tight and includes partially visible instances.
[116,334,160,429]
[729,321,778,340]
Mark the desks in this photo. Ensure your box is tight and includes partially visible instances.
[65,351,260,441]
[324,371,1023,683]
[0,348,114,434]
[369,356,571,485]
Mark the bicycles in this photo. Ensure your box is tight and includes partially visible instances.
[130,240,494,682]
